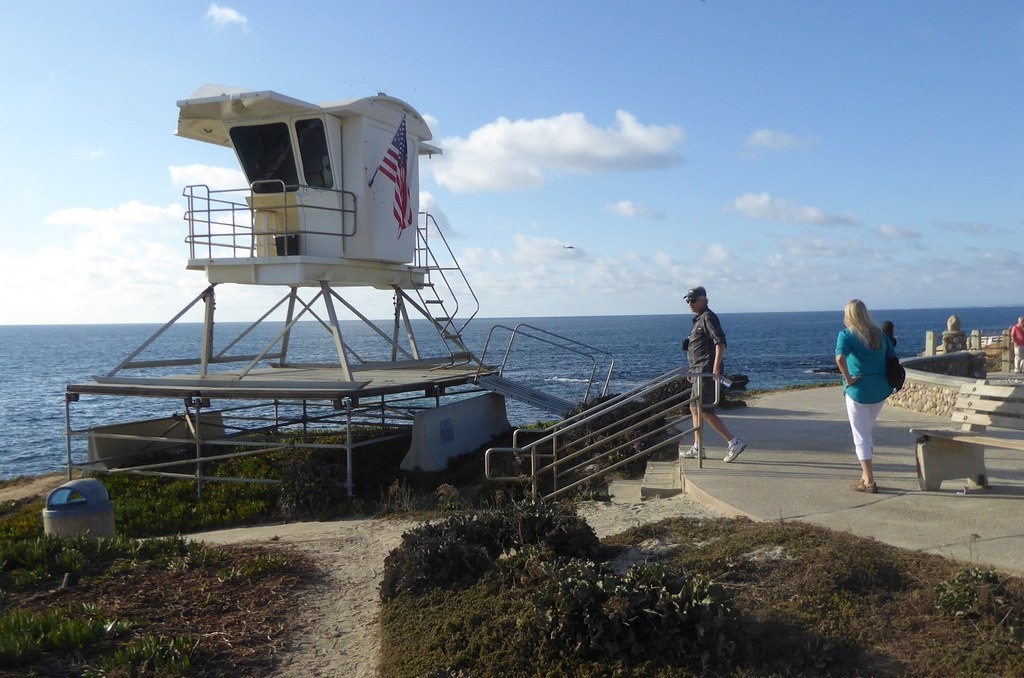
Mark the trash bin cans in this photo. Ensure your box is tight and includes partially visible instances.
[273,234,299,256]
[43,478,115,545]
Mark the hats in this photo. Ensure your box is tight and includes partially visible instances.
[683,287,706,299]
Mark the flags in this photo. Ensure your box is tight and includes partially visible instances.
[379,115,413,239]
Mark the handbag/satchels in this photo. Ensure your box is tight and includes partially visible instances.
[880,333,905,392]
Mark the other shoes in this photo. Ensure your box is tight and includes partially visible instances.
[849,479,878,493]
[1015,370,1020,374]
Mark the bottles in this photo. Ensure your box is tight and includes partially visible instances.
[718,374,732,387]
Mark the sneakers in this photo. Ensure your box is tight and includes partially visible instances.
[724,440,746,462]
[679,447,706,459]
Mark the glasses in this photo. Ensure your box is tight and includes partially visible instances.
[686,295,701,304]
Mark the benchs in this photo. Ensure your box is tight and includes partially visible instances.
[908,378,1024,492]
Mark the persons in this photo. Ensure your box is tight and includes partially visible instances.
[1011,317,1024,373]
[680,287,747,464]
[835,299,897,494]
